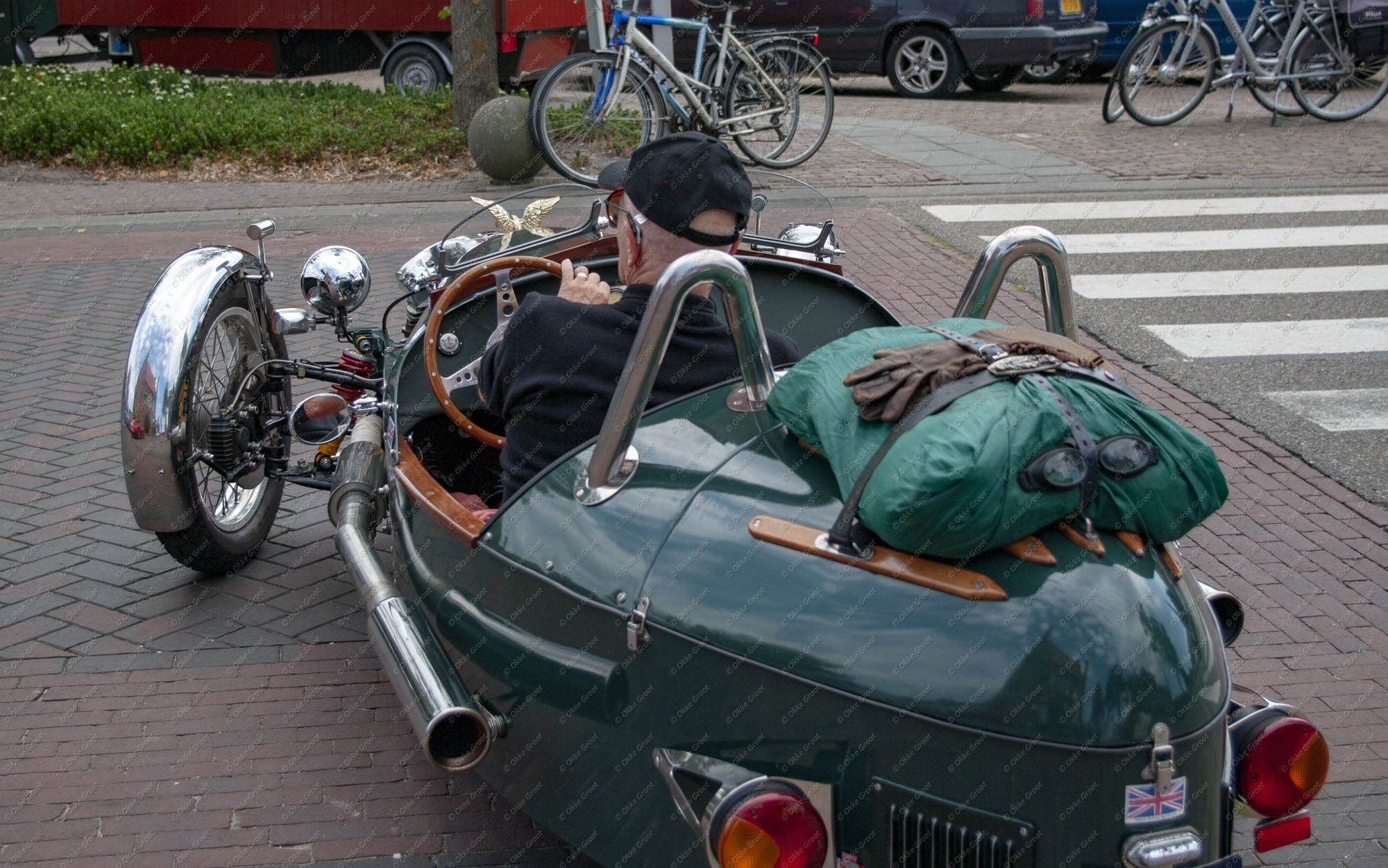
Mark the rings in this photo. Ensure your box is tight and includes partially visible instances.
[576,272,588,280]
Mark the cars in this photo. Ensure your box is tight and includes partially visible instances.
[623,0,1114,102]
[112,154,1333,868]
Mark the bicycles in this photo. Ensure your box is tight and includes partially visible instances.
[1102,0,1388,130]
[528,0,838,191]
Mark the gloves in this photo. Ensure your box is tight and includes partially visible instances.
[843,328,1107,422]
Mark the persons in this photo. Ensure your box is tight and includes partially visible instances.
[479,131,800,506]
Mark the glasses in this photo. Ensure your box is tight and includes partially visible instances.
[605,188,641,244]
[1016,435,1160,492]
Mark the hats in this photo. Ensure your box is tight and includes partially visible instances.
[600,131,753,246]
